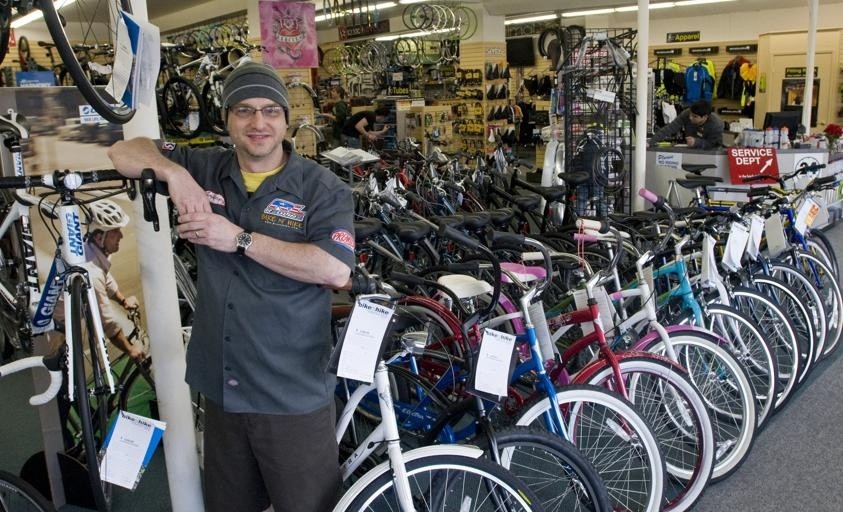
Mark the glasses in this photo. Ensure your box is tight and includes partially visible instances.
[226,104,285,121]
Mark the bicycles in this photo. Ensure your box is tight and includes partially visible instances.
[0,112,161,511]
[0,0,270,142]
[55,305,199,459]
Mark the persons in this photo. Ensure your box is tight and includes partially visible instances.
[106,58,357,511]
[649,100,723,150]
[338,107,390,151]
[43,198,143,448]
[320,86,347,142]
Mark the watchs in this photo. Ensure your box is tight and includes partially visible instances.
[235,228,253,258]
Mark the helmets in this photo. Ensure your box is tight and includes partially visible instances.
[83,201,131,234]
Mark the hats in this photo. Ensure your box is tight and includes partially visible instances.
[221,59,290,127]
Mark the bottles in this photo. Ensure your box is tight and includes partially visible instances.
[766,126,790,150]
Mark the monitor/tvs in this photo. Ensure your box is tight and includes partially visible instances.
[761,112,802,150]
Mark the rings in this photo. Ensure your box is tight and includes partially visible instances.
[194,230,199,238]
